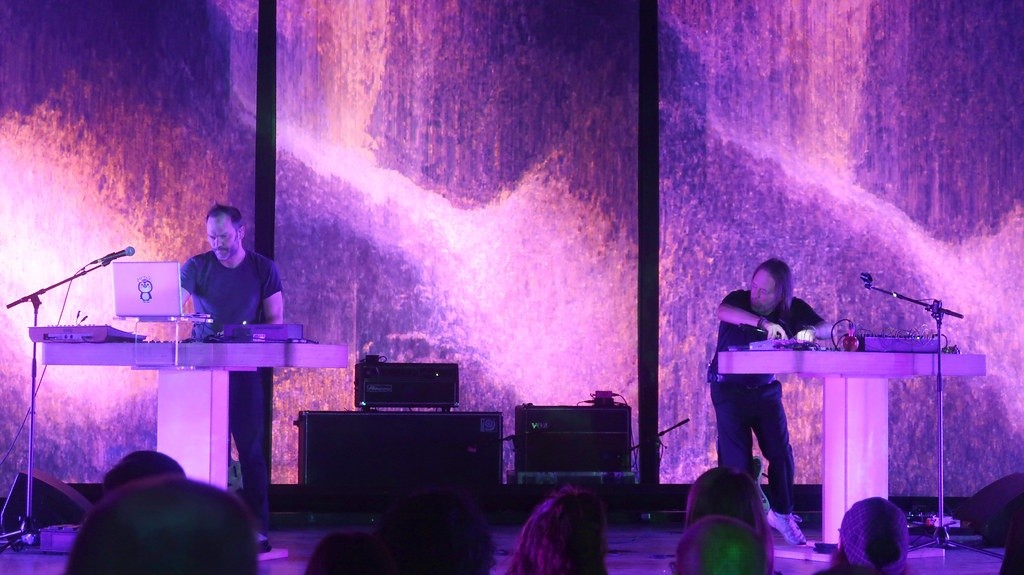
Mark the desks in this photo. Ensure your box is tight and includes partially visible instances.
[717,352,988,563]
[44,342,349,561]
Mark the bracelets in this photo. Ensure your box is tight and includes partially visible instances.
[805,325,816,330]
[757,318,766,327]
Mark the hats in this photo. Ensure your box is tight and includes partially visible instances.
[841,497,908,575]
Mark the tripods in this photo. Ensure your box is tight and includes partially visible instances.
[0,262,111,555]
[858,272,1006,563]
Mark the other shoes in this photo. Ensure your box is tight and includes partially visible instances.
[255,535,271,553]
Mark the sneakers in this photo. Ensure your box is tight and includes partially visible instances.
[767,507,807,547]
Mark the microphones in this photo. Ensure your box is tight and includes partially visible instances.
[90,247,135,264]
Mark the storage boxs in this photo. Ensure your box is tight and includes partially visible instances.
[296,411,503,485]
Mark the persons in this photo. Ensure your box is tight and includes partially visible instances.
[669,468,776,575]
[814,497,908,575]
[66,477,258,575]
[182,205,283,550]
[707,259,835,547]
[302,486,607,575]
[998,504,1024,575]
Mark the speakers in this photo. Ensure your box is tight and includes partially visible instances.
[512,404,632,475]
[960,472,1024,548]
[1,468,95,548]
[297,409,504,491]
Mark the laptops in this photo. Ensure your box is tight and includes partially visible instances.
[112,261,211,319]
